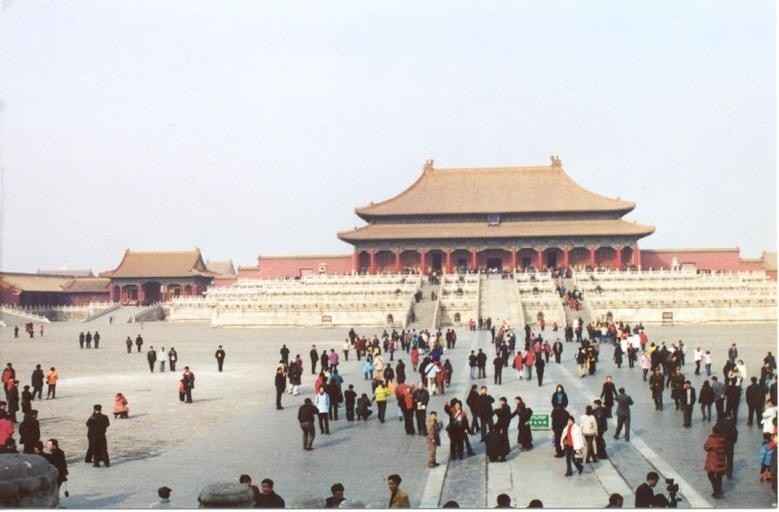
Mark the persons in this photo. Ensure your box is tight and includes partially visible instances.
[1,299,776,508]
[321,261,637,299]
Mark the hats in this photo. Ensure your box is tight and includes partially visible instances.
[159,487,171,493]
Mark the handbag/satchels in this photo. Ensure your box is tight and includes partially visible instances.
[575,453,583,464]
